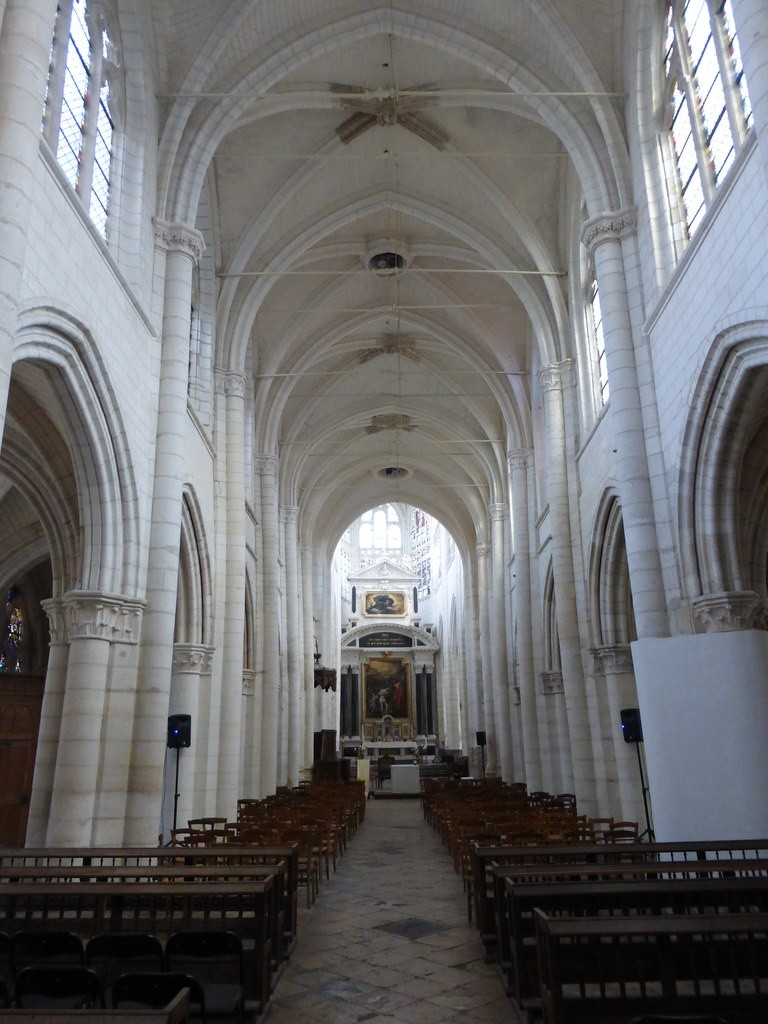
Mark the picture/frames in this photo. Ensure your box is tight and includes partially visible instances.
[362,591,409,618]
[362,657,413,724]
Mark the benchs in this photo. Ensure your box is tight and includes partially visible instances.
[0,846,299,1024]
[472,839,768,1024]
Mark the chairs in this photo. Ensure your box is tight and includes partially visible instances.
[164,930,247,1024]
[86,933,164,1010]
[0,931,13,988]
[14,966,106,1011]
[154,755,661,922]
[111,971,205,1024]
[0,980,11,1010]
[9,928,91,1012]
